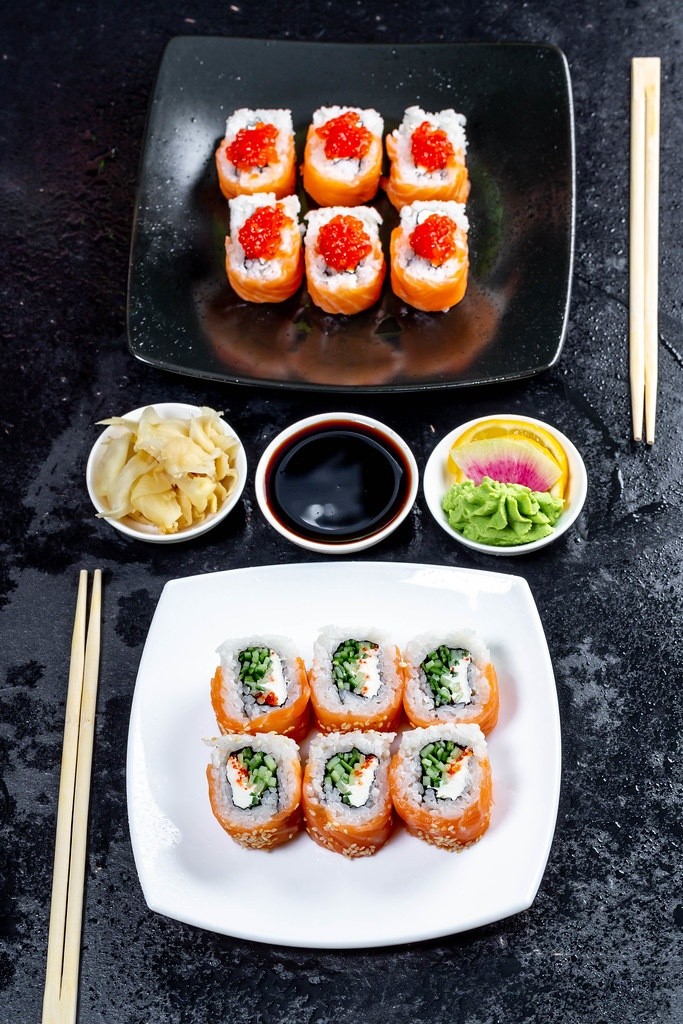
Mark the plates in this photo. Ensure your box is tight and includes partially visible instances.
[86,402,249,544]
[422,414,588,557]
[125,561,561,950]
[255,412,419,554]
[126,34,578,392]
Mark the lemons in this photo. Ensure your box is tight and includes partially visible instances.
[447,418,570,511]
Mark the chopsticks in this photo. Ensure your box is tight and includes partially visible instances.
[42,568,104,1024]
[628,56,661,444]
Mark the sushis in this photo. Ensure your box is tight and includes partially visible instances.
[205,635,500,861]
[215,104,470,314]
